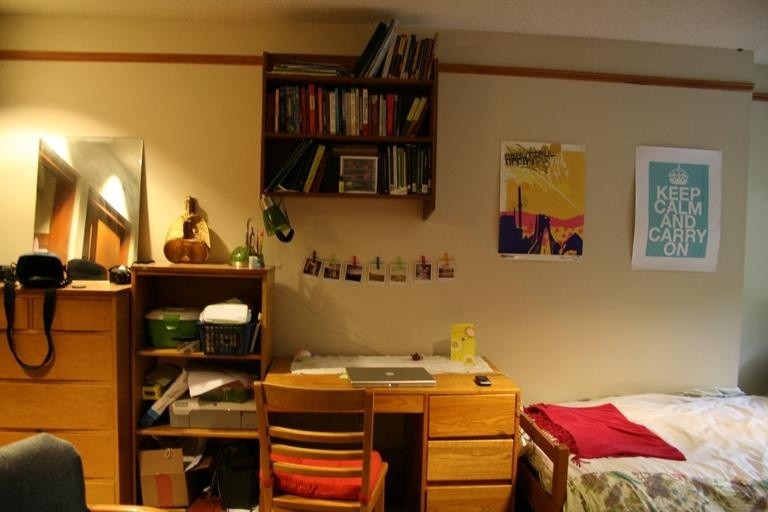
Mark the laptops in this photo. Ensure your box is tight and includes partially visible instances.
[346,367,436,388]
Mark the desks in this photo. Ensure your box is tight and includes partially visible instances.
[263,356,520,511]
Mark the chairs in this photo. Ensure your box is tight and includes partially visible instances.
[0,433,91,511]
[253,379,401,512]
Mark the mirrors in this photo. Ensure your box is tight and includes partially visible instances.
[32,137,143,279]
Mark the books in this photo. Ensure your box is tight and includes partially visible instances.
[261,15,442,202]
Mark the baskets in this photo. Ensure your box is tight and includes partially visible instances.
[196,321,257,356]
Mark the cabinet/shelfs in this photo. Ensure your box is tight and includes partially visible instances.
[0,291,134,504]
[258,51,437,220]
[129,264,274,512]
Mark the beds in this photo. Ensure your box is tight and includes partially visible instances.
[522,384,767,512]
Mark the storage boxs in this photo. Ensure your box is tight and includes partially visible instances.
[140,447,212,508]
[145,307,202,349]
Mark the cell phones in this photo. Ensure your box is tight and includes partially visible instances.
[475,375,491,386]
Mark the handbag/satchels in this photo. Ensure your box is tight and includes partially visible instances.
[3,252,73,370]
